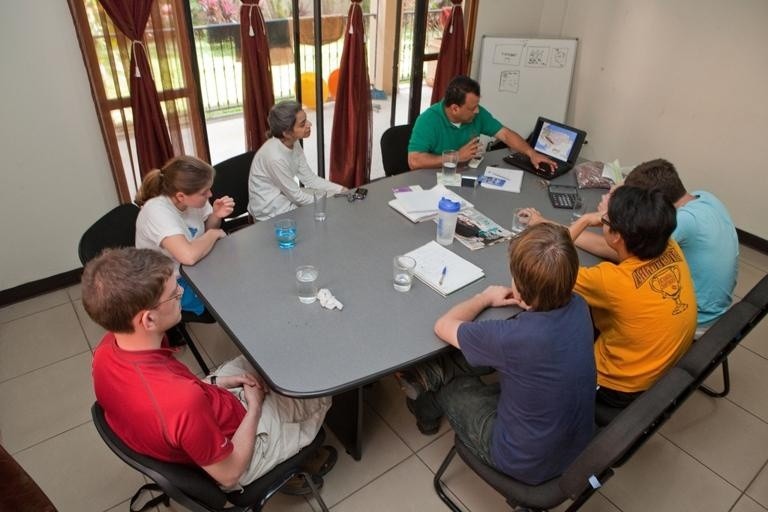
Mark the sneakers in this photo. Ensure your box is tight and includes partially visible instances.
[394,369,427,401]
[276,474,323,497]
[406,395,441,436]
[299,445,338,478]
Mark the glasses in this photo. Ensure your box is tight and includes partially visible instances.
[139,284,185,327]
[599,211,617,235]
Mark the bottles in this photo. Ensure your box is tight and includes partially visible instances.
[434,196,462,246]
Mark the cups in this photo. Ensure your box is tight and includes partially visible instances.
[273,215,299,249]
[392,254,416,294]
[312,190,329,223]
[442,149,459,182]
[512,204,532,232]
[572,196,587,223]
[295,264,319,305]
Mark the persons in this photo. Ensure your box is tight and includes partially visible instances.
[609,158,740,341]
[394,222,597,486]
[517,183,698,409]
[407,74,558,173]
[247,101,350,223]
[135,154,236,351]
[80,246,338,496]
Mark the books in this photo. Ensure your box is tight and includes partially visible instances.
[398,238,486,298]
[388,166,524,251]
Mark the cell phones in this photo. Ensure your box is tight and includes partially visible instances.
[468,156,484,168]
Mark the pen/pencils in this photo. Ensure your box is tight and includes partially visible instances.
[439,266,446,284]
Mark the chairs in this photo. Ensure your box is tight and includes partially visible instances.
[208,149,257,238]
[78,203,212,376]
[91,402,326,512]
[381,123,413,177]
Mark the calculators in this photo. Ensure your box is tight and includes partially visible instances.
[547,185,582,209]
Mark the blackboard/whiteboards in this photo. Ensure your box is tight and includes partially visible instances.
[478,35,578,151]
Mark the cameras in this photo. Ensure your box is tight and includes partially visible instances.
[355,187,367,198]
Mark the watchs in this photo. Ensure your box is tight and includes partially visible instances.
[211,375,217,385]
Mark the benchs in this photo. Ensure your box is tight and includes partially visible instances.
[433,275,768,511]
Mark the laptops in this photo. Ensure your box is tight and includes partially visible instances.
[503,117,586,180]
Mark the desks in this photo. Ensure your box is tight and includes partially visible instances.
[180,134,656,462]
